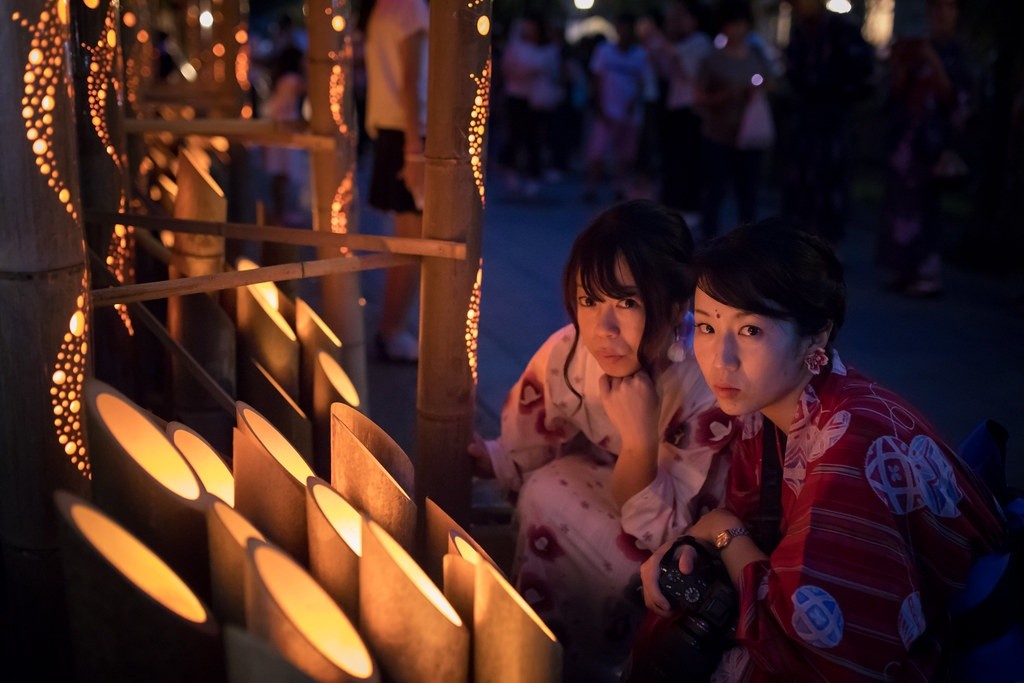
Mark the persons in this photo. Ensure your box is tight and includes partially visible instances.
[467,200,849,683]
[246,15,309,246]
[500,0,1024,299]
[640,225,1024,683]
[364,0,429,366]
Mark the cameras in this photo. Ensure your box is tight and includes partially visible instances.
[656,562,742,651]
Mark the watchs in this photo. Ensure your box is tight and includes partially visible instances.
[712,525,754,551]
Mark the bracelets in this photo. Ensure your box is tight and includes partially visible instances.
[405,150,425,163]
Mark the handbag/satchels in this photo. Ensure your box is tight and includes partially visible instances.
[656,535,740,643]
